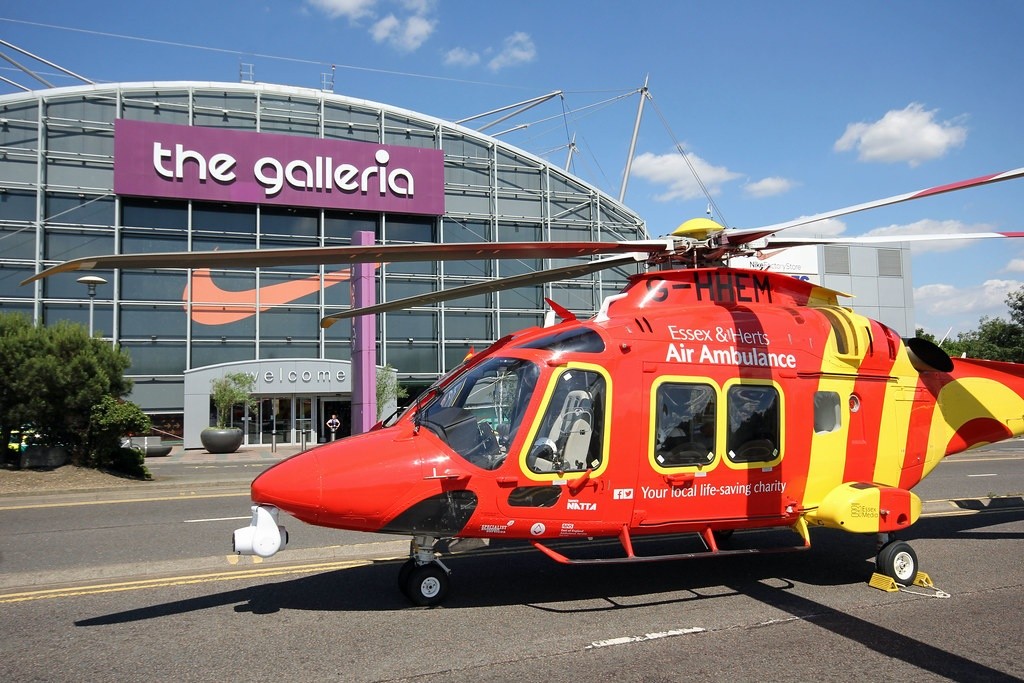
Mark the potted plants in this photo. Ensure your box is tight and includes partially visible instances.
[201,370,259,454]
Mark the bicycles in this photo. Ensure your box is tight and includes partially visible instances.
[120,433,142,451]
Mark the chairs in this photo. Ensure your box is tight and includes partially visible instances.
[505,391,555,451]
[534,390,594,471]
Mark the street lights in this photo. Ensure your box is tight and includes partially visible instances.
[76,276,108,339]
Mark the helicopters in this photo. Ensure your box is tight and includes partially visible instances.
[19,168,1024,604]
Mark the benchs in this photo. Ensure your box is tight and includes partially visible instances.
[121,436,172,457]
